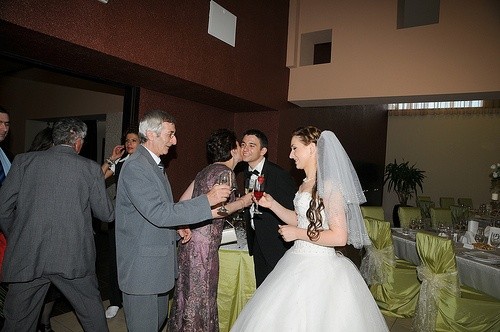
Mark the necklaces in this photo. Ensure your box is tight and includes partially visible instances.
[303,176,316,183]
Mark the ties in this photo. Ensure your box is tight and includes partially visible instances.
[0,147,11,177]
[158,162,165,178]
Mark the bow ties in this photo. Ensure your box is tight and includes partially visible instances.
[247,170,260,179]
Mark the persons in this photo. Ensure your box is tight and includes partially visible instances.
[0,111,12,188]
[0,128,53,332]
[228,126,391,332]
[99,129,141,318]
[116,110,233,332]
[0,118,118,332]
[163,126,254,332]
[235,129,302,289]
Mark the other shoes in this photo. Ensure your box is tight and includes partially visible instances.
[105,305,119,318]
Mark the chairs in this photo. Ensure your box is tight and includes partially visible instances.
[415,232,500,331]
[364,217,421,318]
[397,196,474,232]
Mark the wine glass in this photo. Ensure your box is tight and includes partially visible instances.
[418,219,424,229]
[490,233,499,254]
[446,225,451,238]
[254,178,264,214]
[245,178,253,194]
[440,223,445,237]
[217,170,231,213]
[411,218,416,231]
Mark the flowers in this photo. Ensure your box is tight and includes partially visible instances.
[258,174,264,184]
[489,163,500,194]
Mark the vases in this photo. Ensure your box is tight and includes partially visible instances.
[491,194,498,201]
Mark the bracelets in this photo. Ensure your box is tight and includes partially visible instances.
[105,158,114,166]
[236,196,246,211]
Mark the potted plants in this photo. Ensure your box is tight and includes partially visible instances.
[382,158,428,228]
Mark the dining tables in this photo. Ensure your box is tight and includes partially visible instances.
[469,213,499,227]
[163,227,257,332]
[392,228,500,300]
